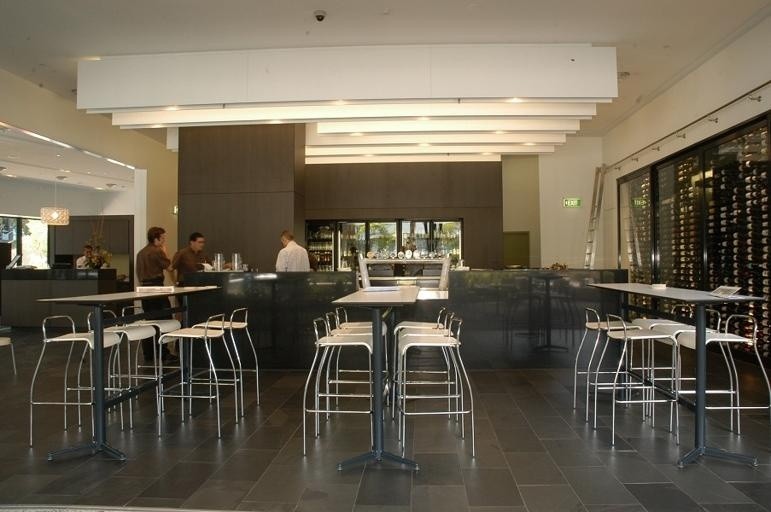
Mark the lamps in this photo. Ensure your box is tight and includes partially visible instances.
[39,169,70,226]
[604,92,762,174]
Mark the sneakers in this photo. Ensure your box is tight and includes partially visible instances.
[162,354,177,360]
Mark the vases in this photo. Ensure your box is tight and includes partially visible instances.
[88,258,104,268]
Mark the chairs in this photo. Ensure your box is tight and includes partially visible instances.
[498,283,577,359]
[302,304,476,459]
[1,302,261,453]
[571,303,771,448]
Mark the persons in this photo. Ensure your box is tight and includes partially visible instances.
[136,226,177,360]
[76,246,95,271]
[275,231,310,272]
[168,232,205,353]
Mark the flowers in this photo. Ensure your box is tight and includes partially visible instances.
[82,231,113,268]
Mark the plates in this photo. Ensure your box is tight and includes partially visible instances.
[504,263,524,268]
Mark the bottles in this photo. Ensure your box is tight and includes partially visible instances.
[313,251,332,270]
[233,250,241,271]
[215,249,223,272]
[341,248,360,268]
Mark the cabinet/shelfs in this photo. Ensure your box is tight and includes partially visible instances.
[358,258,450,291]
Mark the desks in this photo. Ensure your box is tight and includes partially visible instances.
[38,282,224,463]
[523,272,570,353]
[331,284,423,474]
[587,278,765,471]
